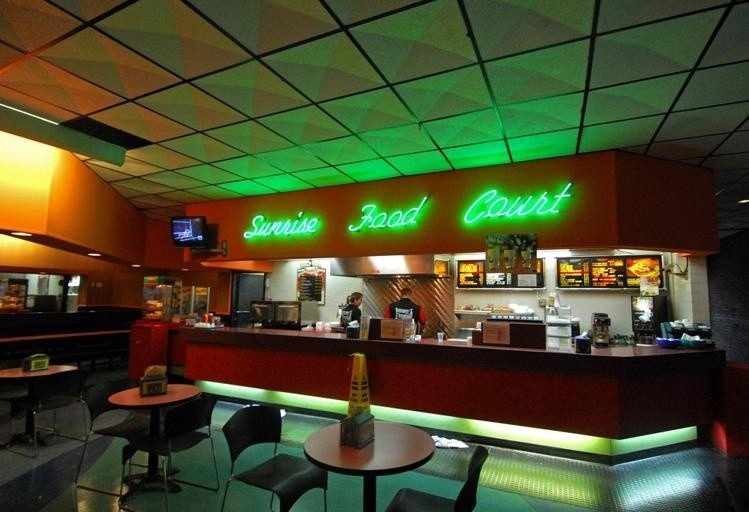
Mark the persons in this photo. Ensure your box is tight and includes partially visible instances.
[384,288,428,335]
[340,292,363,331]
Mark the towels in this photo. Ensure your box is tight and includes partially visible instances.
[430,434,470,451]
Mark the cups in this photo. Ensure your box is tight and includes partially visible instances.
[521,251,531,268]
[504,250,515,269]
[359,314,371,340]
[208,313,213,324]
[437,332,443,342]
[202,313,208,323]
[487,248,499,268]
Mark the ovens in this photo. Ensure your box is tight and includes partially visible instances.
[25,294,56,311]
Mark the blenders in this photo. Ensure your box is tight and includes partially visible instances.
[591,312,611,347]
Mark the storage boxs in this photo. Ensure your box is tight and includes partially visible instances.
[482,320,546,346]
[383,321,414,338]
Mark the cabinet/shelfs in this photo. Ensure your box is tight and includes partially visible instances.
[451,303,494,315]
[179,324,726,465]
[129,315,199,380]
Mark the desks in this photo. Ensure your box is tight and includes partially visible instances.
[108,385,200,492]
[304,421,436,512]
[0,365,78,447]
[0,330,132,341]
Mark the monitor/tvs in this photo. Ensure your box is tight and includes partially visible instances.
[171,216,208,247]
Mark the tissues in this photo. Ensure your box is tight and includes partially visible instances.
[574,331,591,354]
[140,365,167,397]
[23,354,49,371]
[472,321,482,344]
[346,320,360,339]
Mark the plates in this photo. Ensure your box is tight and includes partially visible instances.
[656,338,680,348]
[681,339,716,348]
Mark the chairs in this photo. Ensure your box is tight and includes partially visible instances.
[118,393,220,512]
[74,369,165,498]
[220,406,328,512]
[35,357,88,439]
[387,446,488,512]
[8,335,92,458]
